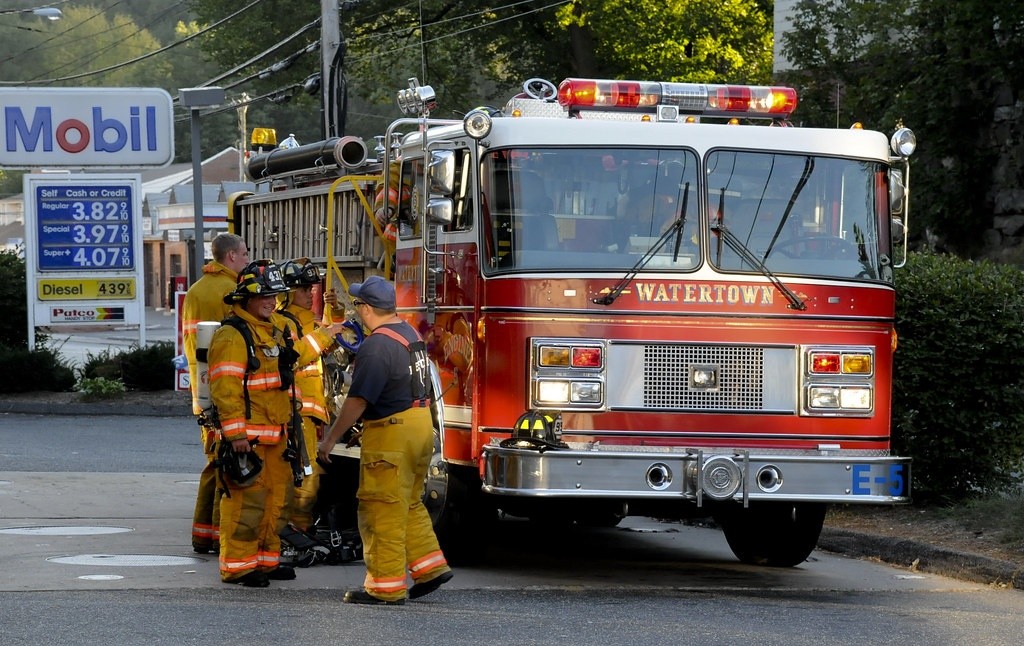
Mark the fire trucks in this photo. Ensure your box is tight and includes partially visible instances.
[225,77,917,566]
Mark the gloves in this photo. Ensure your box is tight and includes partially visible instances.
[376,207,395,228]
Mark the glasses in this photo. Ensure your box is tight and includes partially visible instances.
[353,300,366,306]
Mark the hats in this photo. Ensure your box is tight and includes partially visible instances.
[349,276,396,308]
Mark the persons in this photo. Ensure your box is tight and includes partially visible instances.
[374,157,413,279]
[181,232,349,587]
[318,275,455,605]
[601,187,668,254]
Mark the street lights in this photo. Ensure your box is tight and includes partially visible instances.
[178,86,224,286]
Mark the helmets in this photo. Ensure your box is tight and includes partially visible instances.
[227,259,290,297]
[280,258,322,286]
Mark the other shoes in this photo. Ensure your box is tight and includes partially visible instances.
[343,590,405,605]
[192,544,209,553]
[225,572,270,587]
[409,570,454,599]
[212,543,219,550]
[264,565,296,579]
